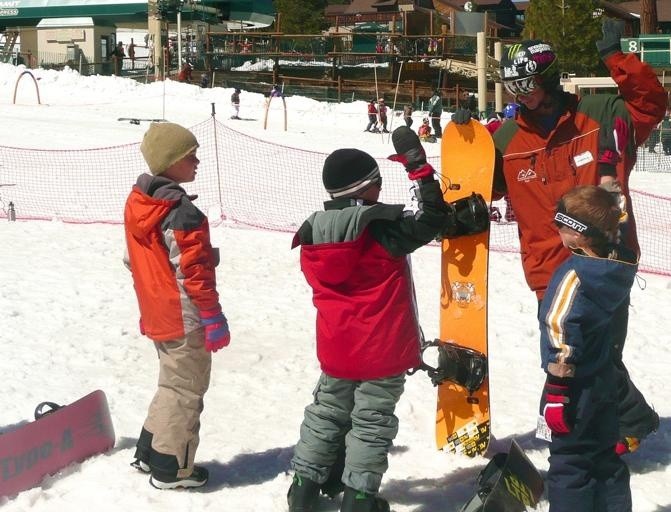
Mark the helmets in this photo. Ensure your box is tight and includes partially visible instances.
[500,40,560,95]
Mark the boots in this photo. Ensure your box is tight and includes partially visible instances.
[288,471,321,512]
[340,485,390,512]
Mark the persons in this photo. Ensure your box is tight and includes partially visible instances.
[177,63,193,84]
[231,86,242,119]
[363,85,443,143]
[539,151,642,512]
[484,15,671,458]
[287,127,447,511]
[270,83,281,98]
[384,38,400,60]
[109,40,126,76]
[123,121,230,490]
[13,54,24,67]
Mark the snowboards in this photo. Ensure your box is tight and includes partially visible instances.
[435,117,496,458]
[0,389,116,497]
[118,117,166,123]
[228,115,255,120]
[481,439,544,512]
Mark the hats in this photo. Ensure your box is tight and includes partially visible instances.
[140,123,199,177]
[323,149,380,201]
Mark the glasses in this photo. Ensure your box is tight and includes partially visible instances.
[504,53,558,97]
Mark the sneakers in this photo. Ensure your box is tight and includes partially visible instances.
[138,459,209,490]
[614,414,659,455]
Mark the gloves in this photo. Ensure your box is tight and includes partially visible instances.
[387,126,435,179]
[596,22,624,60]
[544,381,573,433]
[597,125,619,180]
